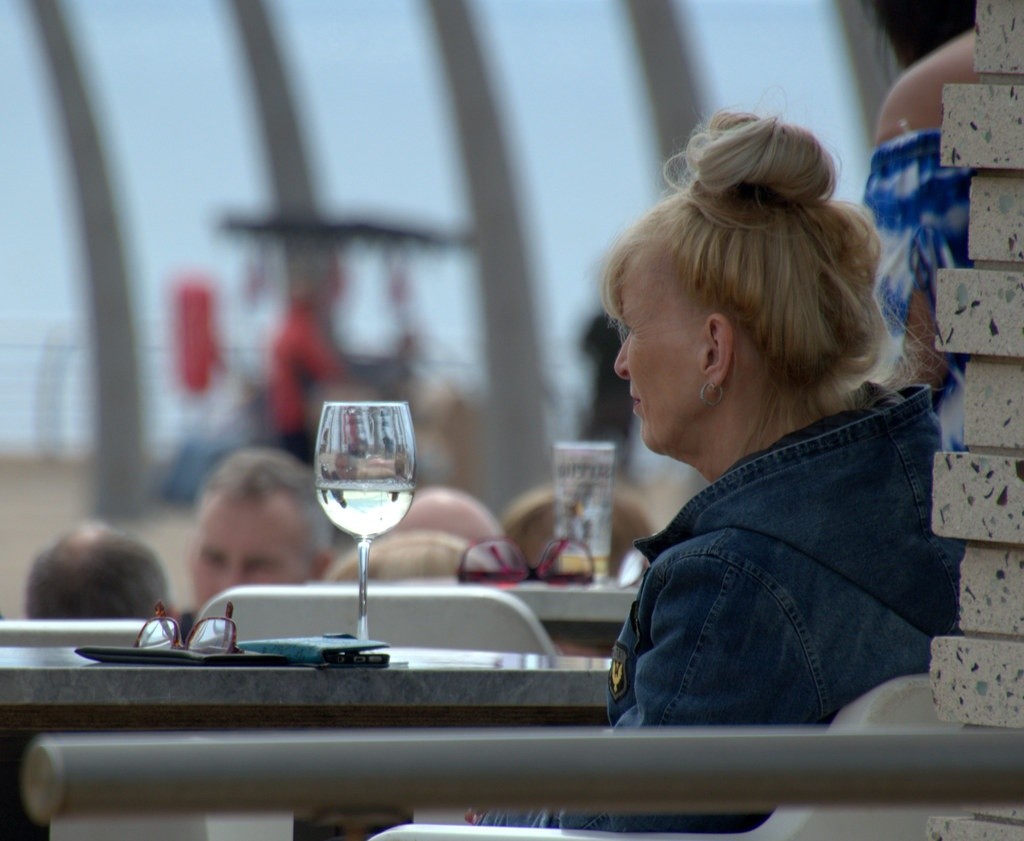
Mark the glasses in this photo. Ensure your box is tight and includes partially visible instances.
[134,600,245,656]
[457,537,596,588]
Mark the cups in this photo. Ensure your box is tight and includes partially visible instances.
[553,443,616,583]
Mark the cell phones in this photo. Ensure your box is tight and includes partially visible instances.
[325,654,391,665]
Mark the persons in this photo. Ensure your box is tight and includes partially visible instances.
[179,446,333,640]
[465,0,981,834]
[387,486,499,548]
[23,524,169,619]
[502,486,654,578]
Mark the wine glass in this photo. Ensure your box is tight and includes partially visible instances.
[314,401,417,640]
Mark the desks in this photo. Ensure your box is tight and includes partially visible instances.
[0,645,613,841]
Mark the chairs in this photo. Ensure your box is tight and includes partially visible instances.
[0,580,942,841]
